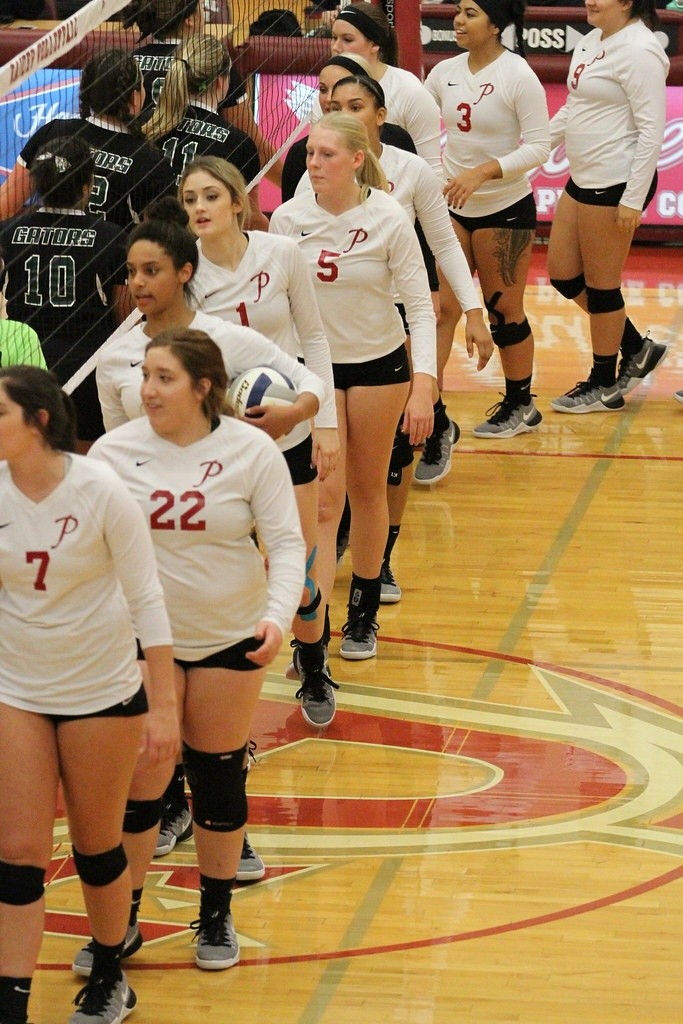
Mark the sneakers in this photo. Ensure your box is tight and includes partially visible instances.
[617,330,668,396]
[68,969,137,1024]
[340,602,380,659]
[290,637,339,727]
[235,832,265,880]
[72,919,144,975]
[380,556,402,603]
[153,793,193,856]
[285,638,332,681]
[473,391,542,438]
[550,367,626,413]
[413,416,461,486]
[189,893,240,969]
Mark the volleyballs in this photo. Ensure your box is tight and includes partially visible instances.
[227,365,299,439]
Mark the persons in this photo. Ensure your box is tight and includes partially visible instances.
[86,157,342,972]
[547,1,669,415]
[422,0,551,438]
[269,0,493,661]
[0,0,289,457]
[1,365,180,1024]
